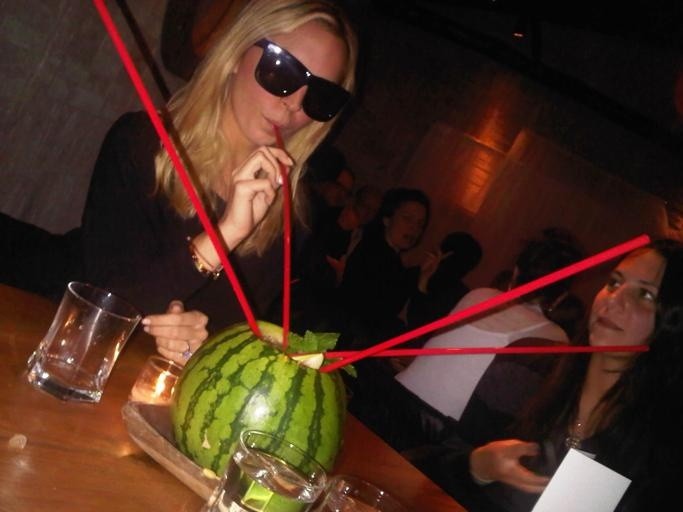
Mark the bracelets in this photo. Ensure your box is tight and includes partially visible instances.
[187,239,222,283]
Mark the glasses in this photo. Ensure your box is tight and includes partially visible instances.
[254,39,350,122]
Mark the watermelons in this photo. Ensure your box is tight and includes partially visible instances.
[170,320,347,512]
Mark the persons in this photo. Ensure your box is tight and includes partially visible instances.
[449,239,683,510]
[61,0,360,377]
[392,227,584,444]
[312,157,488,332]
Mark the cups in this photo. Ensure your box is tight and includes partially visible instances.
[305,472,409,512]
[26,280,143,404]
[200,428,328,511]
[129,355,185,407]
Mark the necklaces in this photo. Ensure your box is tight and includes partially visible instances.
[564,397,593,452]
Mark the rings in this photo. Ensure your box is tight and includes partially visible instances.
[181,342,195,361]
